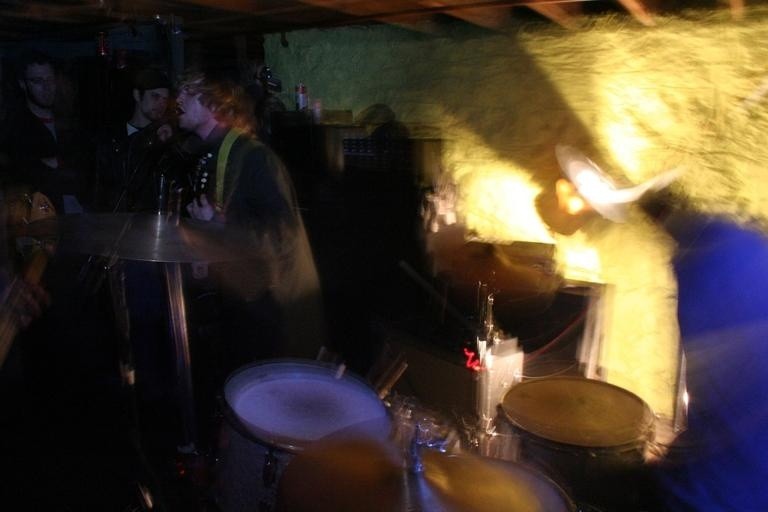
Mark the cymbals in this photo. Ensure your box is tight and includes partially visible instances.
[23,213,294,265]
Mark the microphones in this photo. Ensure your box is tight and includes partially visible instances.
[129,108,177,146]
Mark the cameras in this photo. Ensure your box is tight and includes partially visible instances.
[260,64,281,93]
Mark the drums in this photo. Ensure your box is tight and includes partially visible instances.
[402,454,578,511]
[214,357,398,510]
[496,376,655,497]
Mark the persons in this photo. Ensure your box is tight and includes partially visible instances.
[0,52,324,511]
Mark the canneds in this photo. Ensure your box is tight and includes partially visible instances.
[312,99,320,120]
[295,85,308,112]
